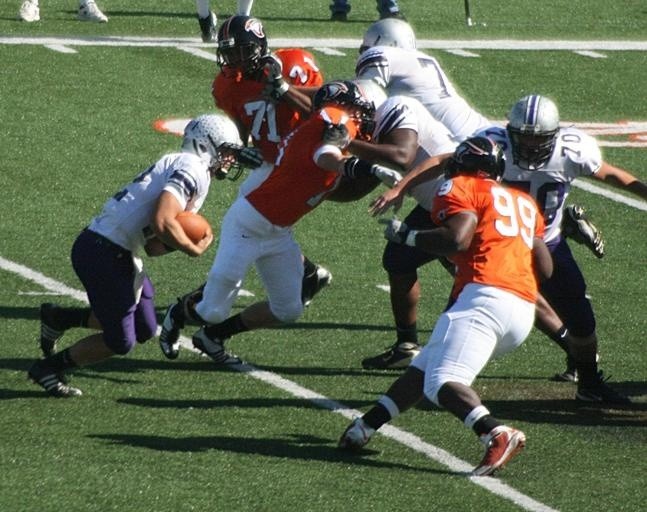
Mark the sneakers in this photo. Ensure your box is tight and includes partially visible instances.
[39,301,69,359]
[337,417,375,453]
[471,423,527,477]
[361,339,423,370]
[300,262,333,306]
[158,302,185,362]
[78,0,109,21]
[563,203,606,258]
[196,5,410,45]
[575,373,632,406]
[25,358,84,398]
[554,352,600,383]
[190,324,243,366]
[15,0,40,22]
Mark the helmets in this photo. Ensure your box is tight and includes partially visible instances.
[503,93,561,171]
[215,15,270,77]
[312,78,376,117]
[318,105,357,141]
[443,136,506,184]
[358,17,418,52]
[180,114,244,182]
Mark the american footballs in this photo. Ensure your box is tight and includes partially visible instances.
[175,213,210,245]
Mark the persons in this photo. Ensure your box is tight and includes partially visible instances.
[329,0,408,24]
[195,0,254,43]
[16,0,109,24]
[29,112,246,397]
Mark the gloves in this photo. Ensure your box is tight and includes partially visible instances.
[322,123,351,150]
[367,162,403,189]
[238,144,264,170]
[255,52,290,99]
[339,156,373,179]
[377,217,416,247]
